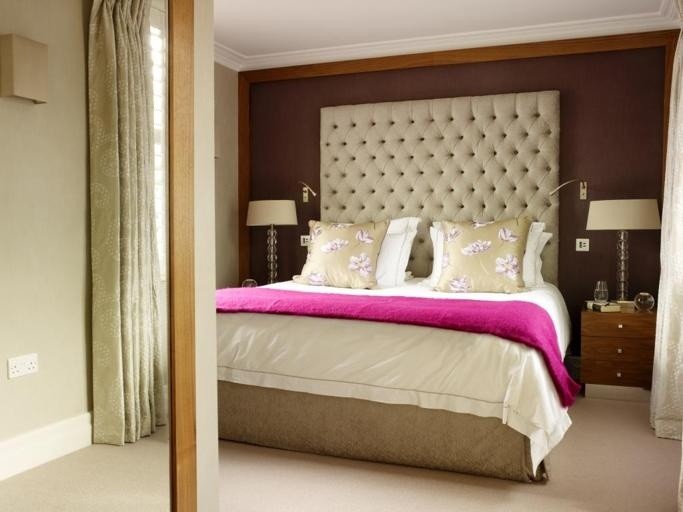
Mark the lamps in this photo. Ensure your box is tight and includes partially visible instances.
[246,200,299,283]
[585,199,661,303]
[298,180,316,203]
[549,178,588,200]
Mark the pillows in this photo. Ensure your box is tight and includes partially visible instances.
[291,216,553,294]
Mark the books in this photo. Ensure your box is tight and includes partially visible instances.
[583,300,621,312]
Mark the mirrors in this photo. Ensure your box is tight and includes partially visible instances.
[1,0,197,511]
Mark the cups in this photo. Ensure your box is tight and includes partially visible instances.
[594,280,609,303]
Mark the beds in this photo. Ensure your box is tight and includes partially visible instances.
[217,279,573,483]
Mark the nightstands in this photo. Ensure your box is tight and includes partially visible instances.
[581,308,657,403]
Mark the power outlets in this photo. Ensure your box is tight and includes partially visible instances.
[576,238,589,252]
[7,353,39,379]
